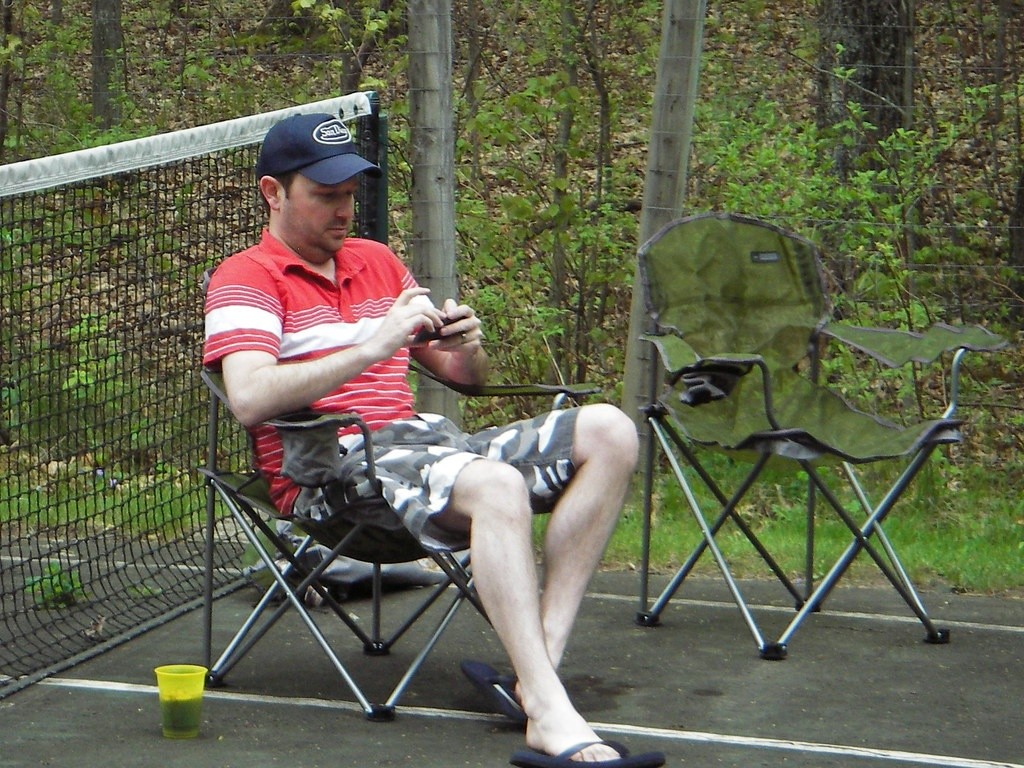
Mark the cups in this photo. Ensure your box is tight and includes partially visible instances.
[154,664,208,739]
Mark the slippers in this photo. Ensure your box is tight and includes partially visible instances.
[460,658,528,721]
[508,739,665,768]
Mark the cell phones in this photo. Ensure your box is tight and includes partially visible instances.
[412,316,468,344]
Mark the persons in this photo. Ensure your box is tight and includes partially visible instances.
[201,112,667,768]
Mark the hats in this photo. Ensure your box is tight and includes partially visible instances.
[255,113,383,185]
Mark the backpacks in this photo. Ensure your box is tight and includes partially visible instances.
[239,517,447,609]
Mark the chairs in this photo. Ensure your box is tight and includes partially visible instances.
[635,213,1013,665]
[191,264,606,728]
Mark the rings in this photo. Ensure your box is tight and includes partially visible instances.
[461,334,468,343]
